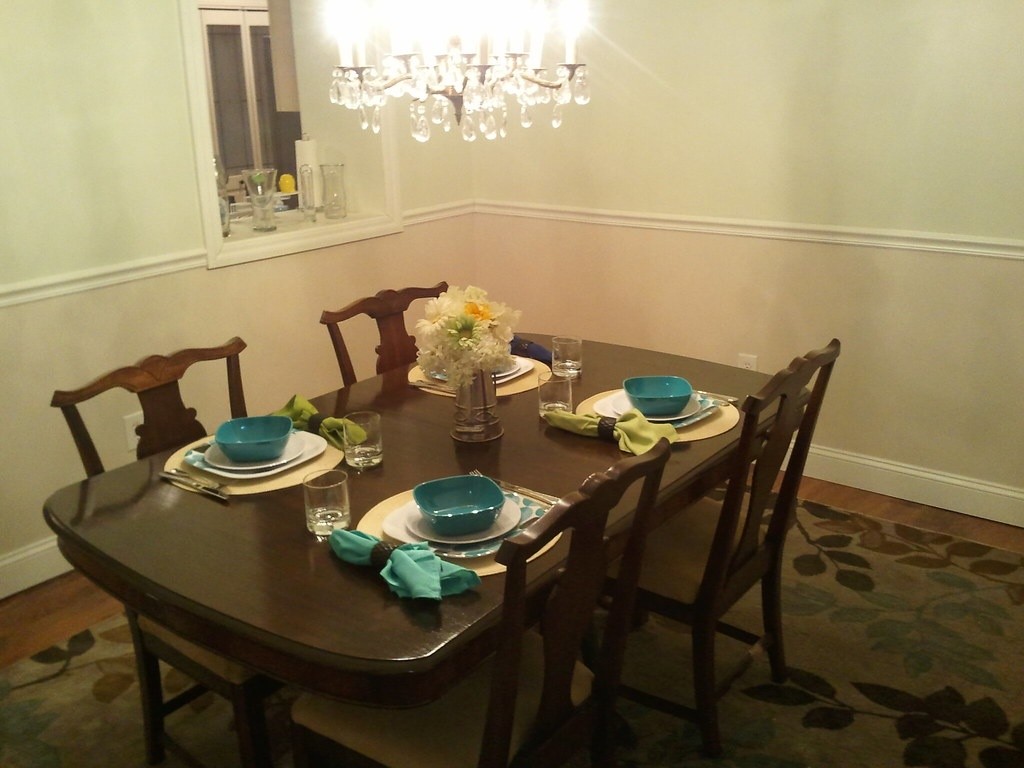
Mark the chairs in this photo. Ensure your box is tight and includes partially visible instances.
[321,282,449,388]
[50,337,292,768]
[538,338,841,754]
[288,437,671,767]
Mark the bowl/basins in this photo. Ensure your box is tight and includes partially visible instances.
[412,474,505,538]
[623,375,693,416]
[214,415,293,463]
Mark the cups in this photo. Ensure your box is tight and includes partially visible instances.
[319,163,348,220]
[342,410,383,471]
[303,469,351,543]
[538,369,572,419]
[551,335,583,378]
[241,168,278,233]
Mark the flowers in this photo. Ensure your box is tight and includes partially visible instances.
[413,286,522,391]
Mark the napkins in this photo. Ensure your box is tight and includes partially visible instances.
[509,336,552,365]
[544,410,679,456]
[328,529,481,600]
[267,394,368,450]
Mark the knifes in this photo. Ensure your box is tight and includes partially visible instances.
[409,381,457,394]
[158,471,229,500]
[491,477,559,505]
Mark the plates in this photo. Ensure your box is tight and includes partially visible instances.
[382,491,546,559]
[610,393,701,421]
[183,428,328,480]
[204,432,307,471]
[492,361,520,378]
[430,355,535,385]
[406,497,522,545]
[592,390,721,429]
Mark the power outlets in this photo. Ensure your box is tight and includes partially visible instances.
[738,353,758,372]
[124,411,144,452]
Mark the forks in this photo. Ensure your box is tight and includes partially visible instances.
[470,469,481,475]
[171,468,229,491]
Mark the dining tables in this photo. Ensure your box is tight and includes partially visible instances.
[43,333,813,748]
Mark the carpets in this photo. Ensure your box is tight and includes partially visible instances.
[0,491,1024,768]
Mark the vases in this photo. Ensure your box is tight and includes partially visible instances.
[450,368,505,442]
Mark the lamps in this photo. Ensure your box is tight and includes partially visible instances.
[330,0,591,142]
